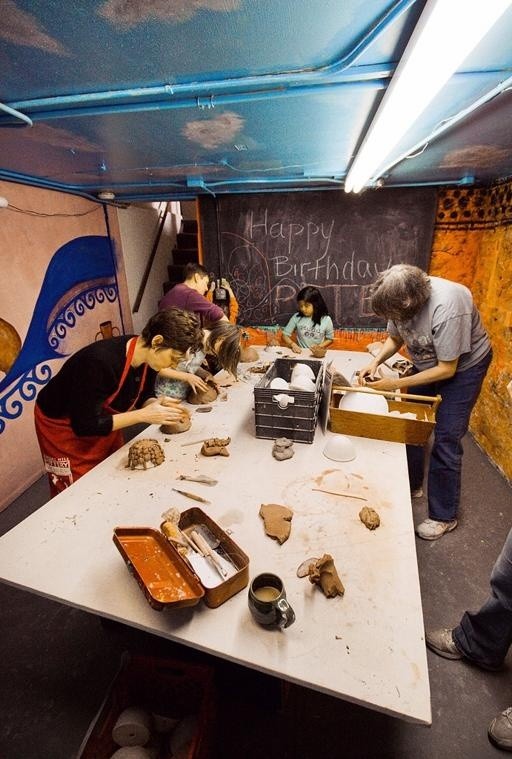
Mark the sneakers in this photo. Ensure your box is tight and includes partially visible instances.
[487,705,511,751]
[427,628,499,672]
[414,520,458,541]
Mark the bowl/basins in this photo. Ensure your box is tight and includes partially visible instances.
[270,363,315,404]
[322,432,355,461]
[240,347,260,363]
[310,346,328,358]
[187,385,218,404]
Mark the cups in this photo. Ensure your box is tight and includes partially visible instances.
[248,572,295,631]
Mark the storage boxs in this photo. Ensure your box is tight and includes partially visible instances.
[253,357,325,444]
[113,507,250,612]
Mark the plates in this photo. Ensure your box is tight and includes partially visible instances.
[338,385,390,417]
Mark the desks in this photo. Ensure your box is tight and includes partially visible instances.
[0,345,435,730]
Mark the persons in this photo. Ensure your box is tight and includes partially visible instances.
[425,529,512,751]
[358,265,493,540]
[282,286,334,354]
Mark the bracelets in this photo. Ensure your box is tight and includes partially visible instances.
[288,341,294,347]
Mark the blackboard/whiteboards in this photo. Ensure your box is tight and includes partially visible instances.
[197,183,438,328]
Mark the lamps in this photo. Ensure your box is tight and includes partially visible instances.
[342,0,512,195]
